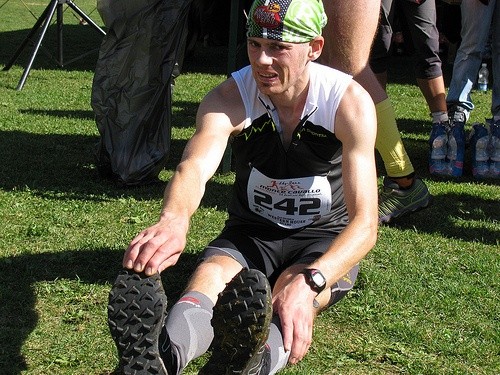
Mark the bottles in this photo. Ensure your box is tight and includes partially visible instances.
[478,63,489,92]
[432,113,500,180]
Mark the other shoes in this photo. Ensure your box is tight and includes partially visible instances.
[196,268,273,375]
[107,264,181,375]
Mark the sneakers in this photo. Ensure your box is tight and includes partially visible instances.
[378,174,432,224]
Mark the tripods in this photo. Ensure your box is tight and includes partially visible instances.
[2,0,107,91]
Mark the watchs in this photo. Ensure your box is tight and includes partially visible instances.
[300,269,326,293]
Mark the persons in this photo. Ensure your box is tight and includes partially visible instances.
[90,0,193,187]
[313,0,500,227]
[107,0,378,375]
